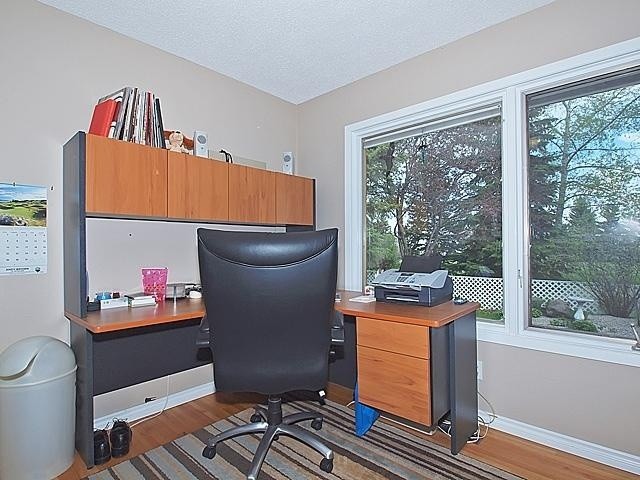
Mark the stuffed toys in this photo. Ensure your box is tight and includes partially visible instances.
[163,129,195,153]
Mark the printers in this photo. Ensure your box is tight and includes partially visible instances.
[372,254,454,307]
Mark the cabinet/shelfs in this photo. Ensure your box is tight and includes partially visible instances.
[63,131,314,227]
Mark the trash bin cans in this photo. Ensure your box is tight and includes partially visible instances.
[0,336,77,480]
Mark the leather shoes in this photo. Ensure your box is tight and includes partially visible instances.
[110,420,132,458]
[94,429,112,466]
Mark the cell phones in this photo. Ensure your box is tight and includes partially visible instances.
[454,297,467,305]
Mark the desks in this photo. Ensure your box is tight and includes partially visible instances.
[568,296,594,320]
[65,288,481,469]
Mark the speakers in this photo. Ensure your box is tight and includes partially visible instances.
[280,152,294,173]
[194,130,210,158]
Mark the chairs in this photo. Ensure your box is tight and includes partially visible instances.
[196,226,345,480]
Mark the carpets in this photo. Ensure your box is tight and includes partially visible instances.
[76,398,529,480]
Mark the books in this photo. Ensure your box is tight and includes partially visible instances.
[88,88,166,149]
[125,292,155,308]
